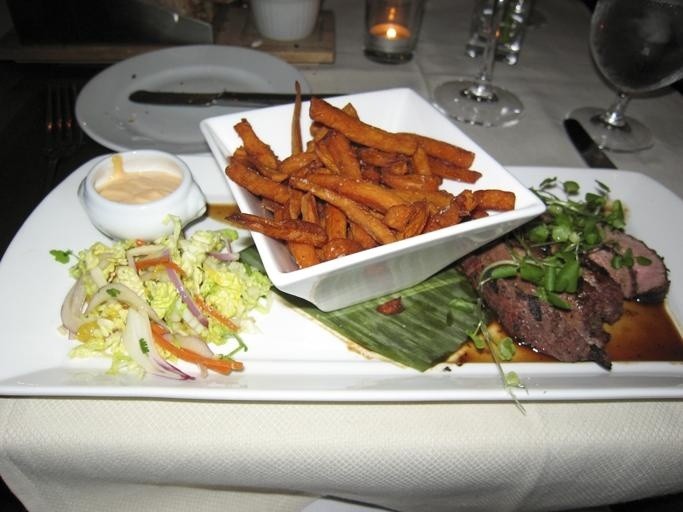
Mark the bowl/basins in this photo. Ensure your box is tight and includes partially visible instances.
[77,149,208,242]
[198,87,548,315]
[249,1,323,43]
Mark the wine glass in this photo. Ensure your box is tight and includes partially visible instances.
[565,1,683,155]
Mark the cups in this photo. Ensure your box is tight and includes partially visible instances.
[467,0,534,67]
[363,1,425,65]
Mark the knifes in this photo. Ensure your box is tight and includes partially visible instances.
[127,88,349,110]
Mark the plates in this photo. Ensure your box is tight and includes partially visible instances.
[74,44,314,157]
[1,153,682,404]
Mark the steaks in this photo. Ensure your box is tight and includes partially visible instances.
[460,206,671,371]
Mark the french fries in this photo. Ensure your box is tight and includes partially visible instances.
[223,80,516,268]
